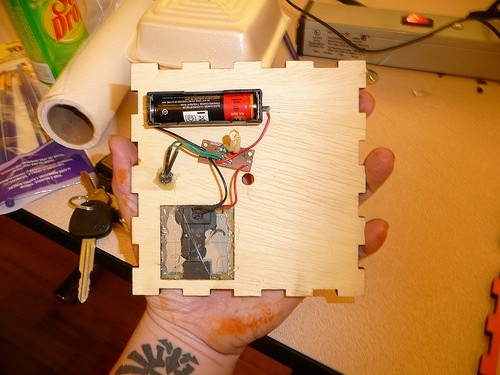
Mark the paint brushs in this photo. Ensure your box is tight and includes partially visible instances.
[0,62,58,208]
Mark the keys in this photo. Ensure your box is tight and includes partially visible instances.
[55,170,129,305]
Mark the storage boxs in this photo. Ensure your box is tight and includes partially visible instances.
[122,0,294,70]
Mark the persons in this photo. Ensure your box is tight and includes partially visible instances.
[108,89,395,375]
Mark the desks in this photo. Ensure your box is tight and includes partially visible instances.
[0,0,500,375]
